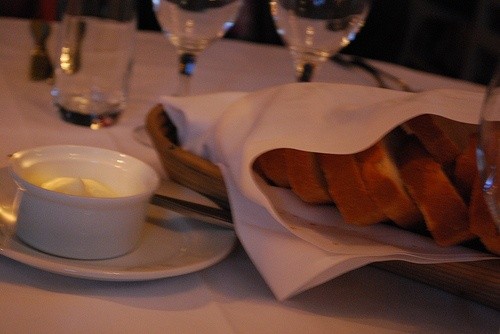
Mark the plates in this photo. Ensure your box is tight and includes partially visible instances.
[0,166,234,279]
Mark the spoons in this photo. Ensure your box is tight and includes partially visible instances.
[29,19,54,80]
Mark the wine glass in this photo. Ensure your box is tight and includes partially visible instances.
[270,0,369,84]
[151,0,242,96]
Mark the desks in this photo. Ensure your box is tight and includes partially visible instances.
[0,15,500,334]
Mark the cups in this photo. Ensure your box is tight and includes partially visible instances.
[50,0,139,127]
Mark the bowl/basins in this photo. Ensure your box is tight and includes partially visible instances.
[5,145,159,260]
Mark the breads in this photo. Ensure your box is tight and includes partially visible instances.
[258,114,500,257]
